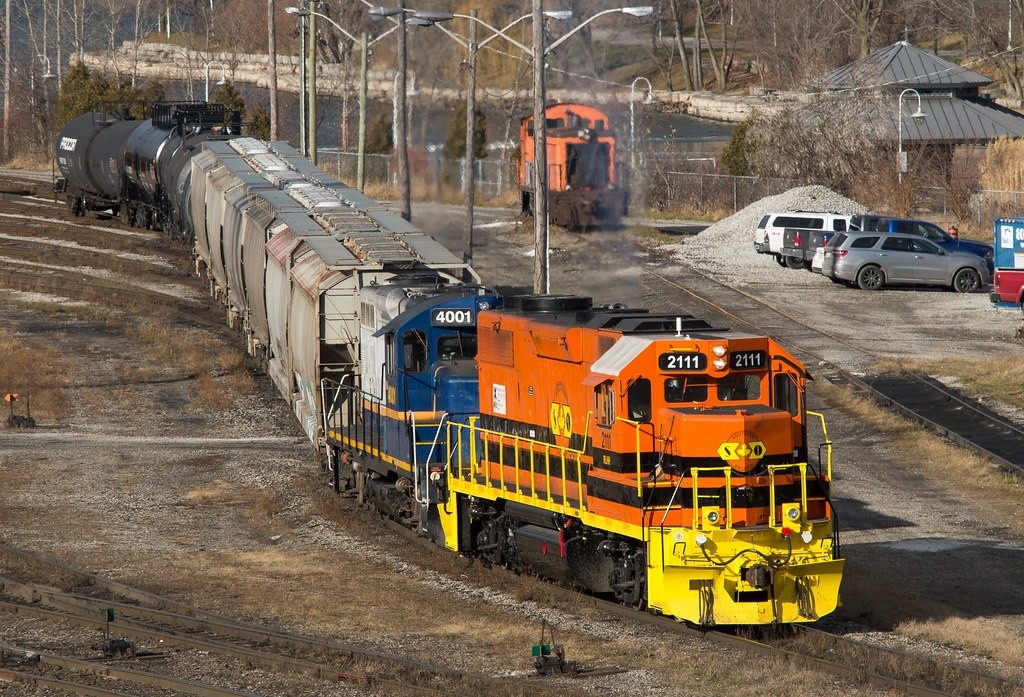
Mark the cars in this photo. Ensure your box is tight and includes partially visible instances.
[753,209,995,294]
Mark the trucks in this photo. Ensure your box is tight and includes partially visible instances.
[989,216,1024,318]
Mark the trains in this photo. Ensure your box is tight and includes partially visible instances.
[512,102,627,230]
[50,94,846,630]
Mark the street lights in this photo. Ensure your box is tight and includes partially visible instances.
[29,54,57,122]
[629,75,656,172]
[204,60,228,104]
[283,6,436,192]
[367,5,574,281]
[896,87,927,188]
[411,5,656,293]
